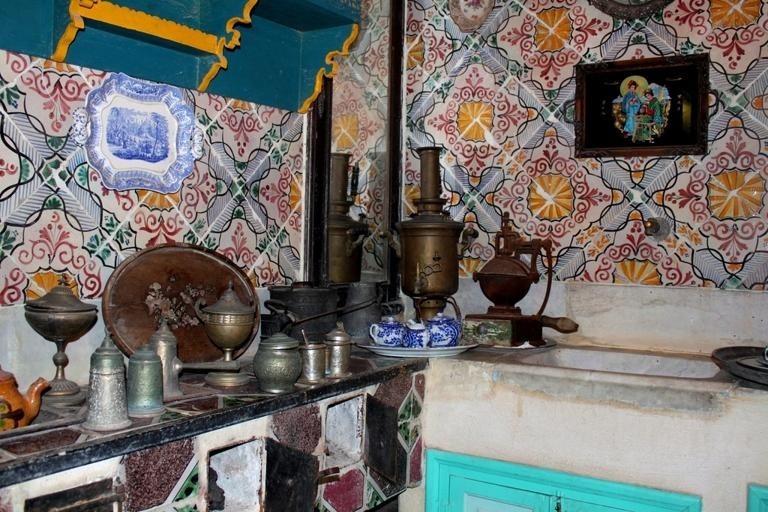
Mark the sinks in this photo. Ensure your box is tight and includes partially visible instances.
[499,343,742,392]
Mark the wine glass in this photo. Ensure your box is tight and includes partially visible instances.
[24,304,96,408]
[200,308,255,387]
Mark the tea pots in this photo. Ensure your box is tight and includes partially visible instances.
[370,313,461,347]
[0,365,50,432]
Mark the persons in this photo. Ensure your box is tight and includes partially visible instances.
[634,88,666,144]
[621,81,642,137]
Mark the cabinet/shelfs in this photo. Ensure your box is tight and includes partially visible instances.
[423,447,703,511]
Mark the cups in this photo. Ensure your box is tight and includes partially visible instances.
[301,339,354,384]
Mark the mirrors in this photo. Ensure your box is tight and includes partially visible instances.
[304,0,405,309]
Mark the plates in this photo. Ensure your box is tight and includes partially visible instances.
[74,70,204,194]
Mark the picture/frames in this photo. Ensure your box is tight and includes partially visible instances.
[574,53,710,158]
[589,0,675,20]
